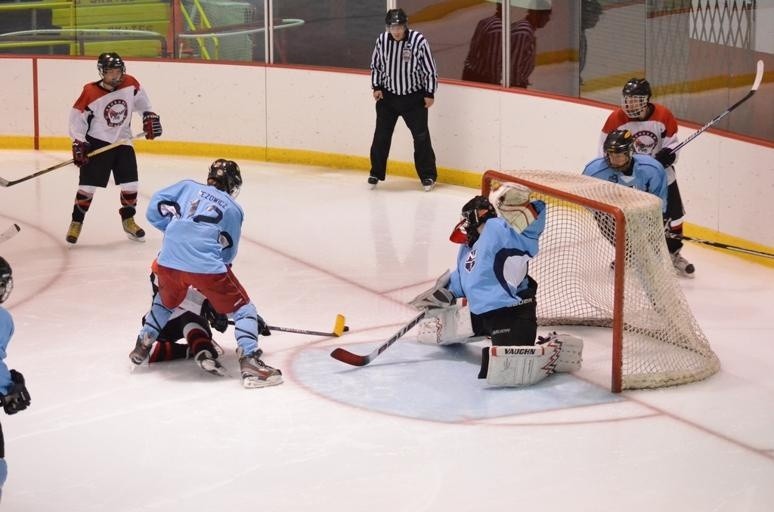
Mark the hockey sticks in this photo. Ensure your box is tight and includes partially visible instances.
[0,132,149,186]
[670,59,764,153]
[331,312,425,366]
[228,314,345,337]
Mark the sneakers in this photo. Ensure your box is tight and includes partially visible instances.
[422,178,434,186]
[368,177,379,184]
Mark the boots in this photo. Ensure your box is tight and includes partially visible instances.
[66,213,84,243]
[670,249,695,274]
[118,205,147,239]
[129,332,157,364]
[196,349,224,376]
[236,346,281,379]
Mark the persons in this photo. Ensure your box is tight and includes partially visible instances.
[146,265,271,378]
[0,255,26,501]
[367,9,438,187]
[417,186,584,386]
[601,78,697,274]
[462,0,603,92]
[582,128,668,268]
[129,158,283,378]
[66,52,163,244]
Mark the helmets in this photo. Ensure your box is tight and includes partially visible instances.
[0,258,14,305]
[603,131,635,170]
[96,51,126,87]
[386,10,406,27]
[206,158,242,200]
[459,196,498,248]
[621,79,652,120]
[581,1,607,14]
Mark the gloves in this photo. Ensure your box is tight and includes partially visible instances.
[1,370,31,416]
[142,112,162,140]
[655,148,676,171]
[257,315,271,337]
[73,139,91,168]
[205,301,228,333]
